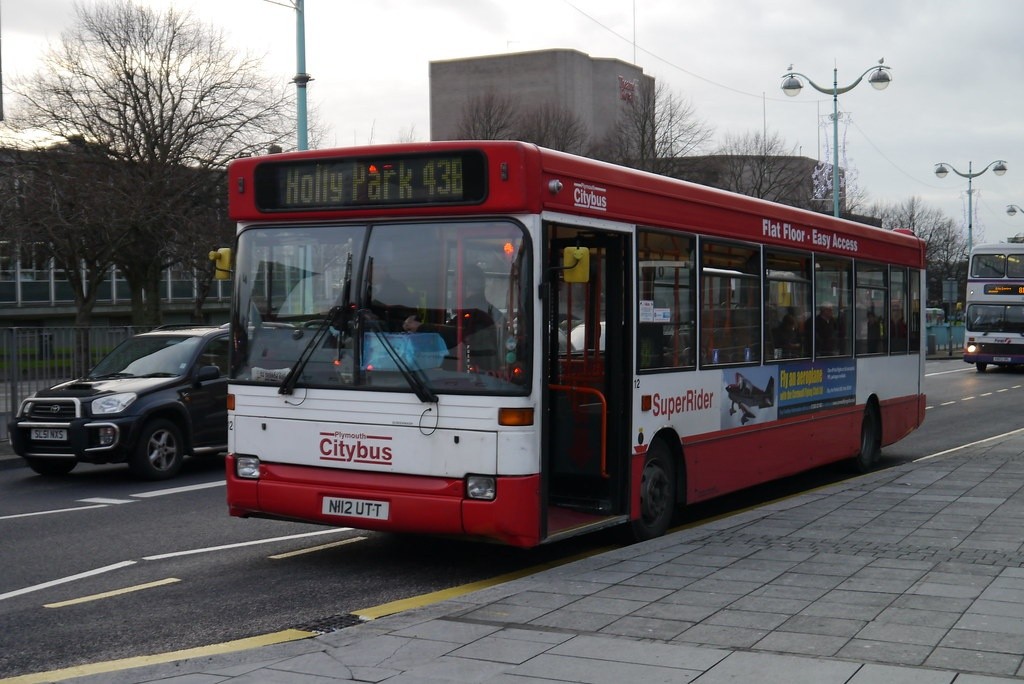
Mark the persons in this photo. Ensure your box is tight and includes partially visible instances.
[772,302,848,359]
[982,308,992,318]
[943,303,950,323]
[360,255,420,331]
[956,301,963,311]
[403,260,509,374]
[866,308,906,352]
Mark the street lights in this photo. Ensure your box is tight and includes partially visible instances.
[779,57,891,217]
[934,160,1007,260]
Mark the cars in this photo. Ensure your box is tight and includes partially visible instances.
[449,308,606,366]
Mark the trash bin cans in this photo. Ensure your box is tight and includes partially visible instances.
[927,334,936,354]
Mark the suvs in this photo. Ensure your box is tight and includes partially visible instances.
[6,323,232,483]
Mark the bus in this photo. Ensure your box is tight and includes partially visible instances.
[926,308,945,328]
[954,243,1024,371]
[208,140,926,549]
